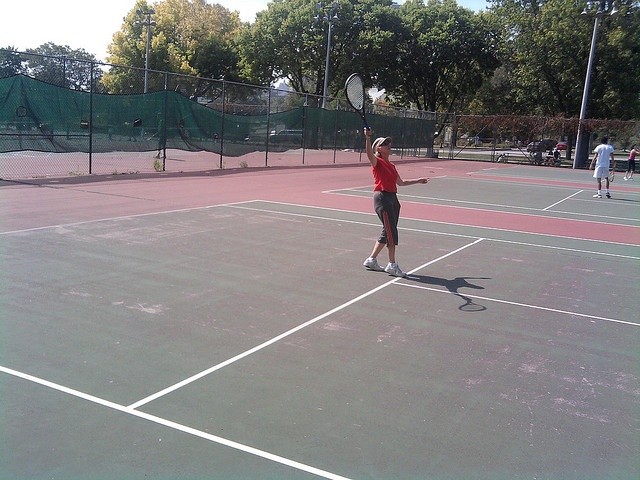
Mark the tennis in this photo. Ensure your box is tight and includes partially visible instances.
[434,131,438,135]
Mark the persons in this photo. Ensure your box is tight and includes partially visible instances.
[624,144,639,181]
[363,127,428,278]
[590,136,615,199]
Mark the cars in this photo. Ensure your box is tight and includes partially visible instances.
[527,142,547,152]
[556,142,572,150]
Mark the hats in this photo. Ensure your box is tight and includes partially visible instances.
[373,137,392,154]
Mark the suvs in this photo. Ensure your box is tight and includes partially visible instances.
[269,130,303,145]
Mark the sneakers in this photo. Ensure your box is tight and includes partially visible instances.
[363,258,384,272]
[384,262,406,277]
[593,193,602,199]
[628,177,634,181]
[606,193,612,199]
[623,177,628,180]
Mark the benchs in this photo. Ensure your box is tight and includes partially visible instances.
[503,153,561,166]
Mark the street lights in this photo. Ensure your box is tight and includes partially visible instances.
[316,2,340,109]
[134,9,156,138]
[572,1,619,169]
